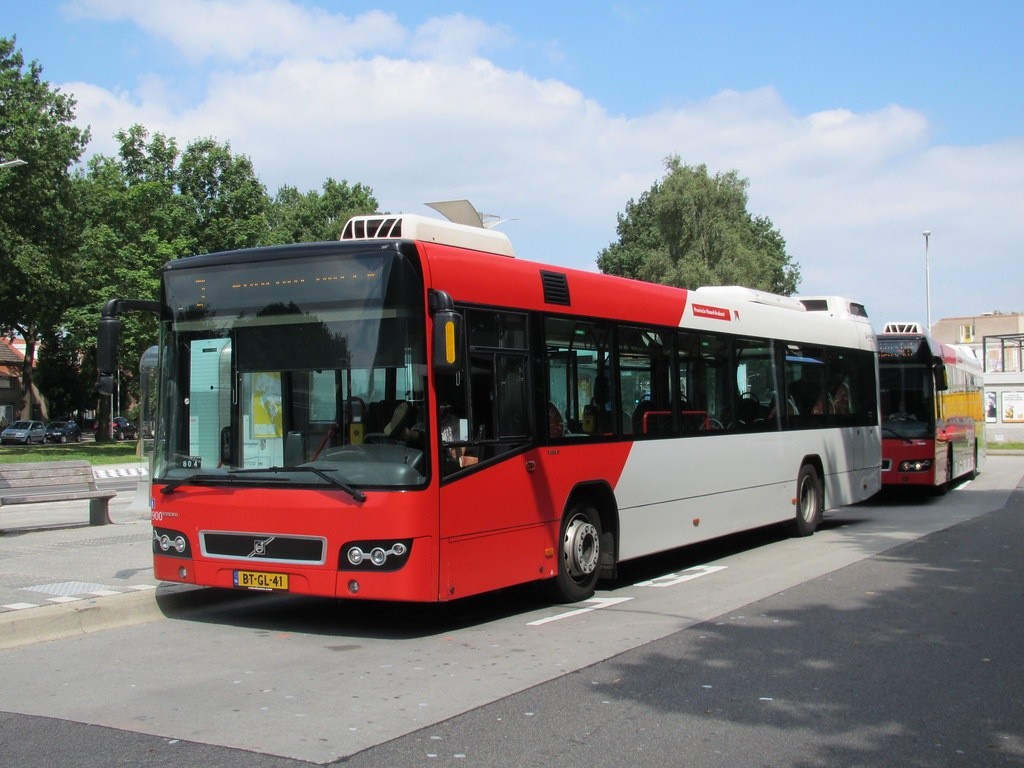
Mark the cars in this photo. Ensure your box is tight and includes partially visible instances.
[0,419,47,445]
[44,421,82,443]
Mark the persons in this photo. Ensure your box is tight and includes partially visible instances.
[383,384,457,463]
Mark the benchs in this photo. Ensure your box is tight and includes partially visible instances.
[0,460,117,526]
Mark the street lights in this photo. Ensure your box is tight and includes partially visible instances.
[922,230,932,336]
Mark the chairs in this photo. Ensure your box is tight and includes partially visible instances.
[633,382,854,435]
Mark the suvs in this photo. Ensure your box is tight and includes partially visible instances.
[93,416,138,440]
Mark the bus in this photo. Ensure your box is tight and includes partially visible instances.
[874,320,985,497]
[97,213,884,604]
